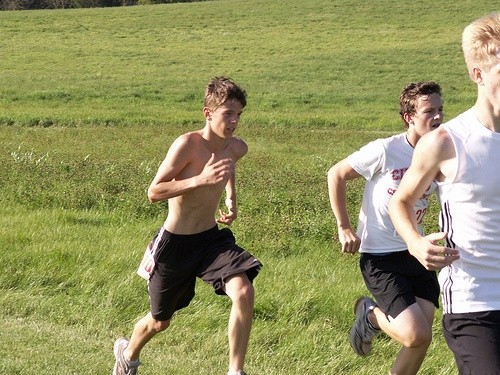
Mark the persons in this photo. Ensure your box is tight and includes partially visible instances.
[326,79,446,375]
[111,75,264,374]
[387,10,500,375]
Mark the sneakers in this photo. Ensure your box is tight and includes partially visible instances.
[350,295,382,358]
[112,338,140,375]
